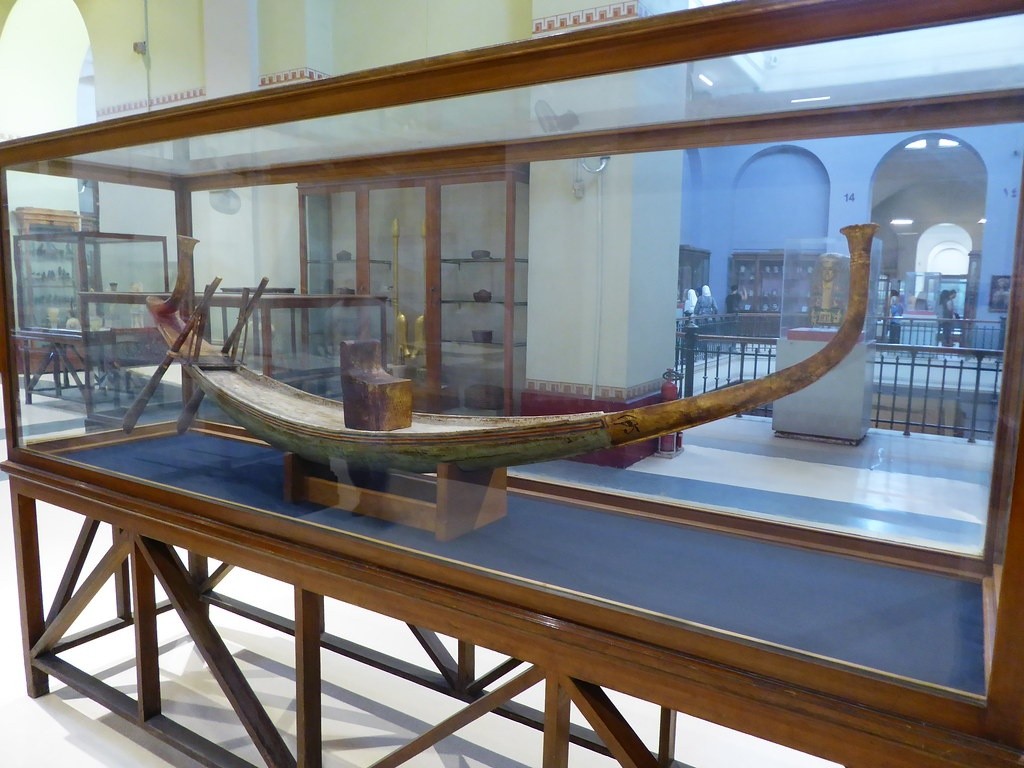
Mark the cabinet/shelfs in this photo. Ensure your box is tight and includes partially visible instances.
[676,247,712,303]
[0,0,1024,768]
[79,291,390,445]
[296,162,530,416]
[729,249,822,313]
[13,230,171,422]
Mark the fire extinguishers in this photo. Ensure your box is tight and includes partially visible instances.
[658,368,684,452]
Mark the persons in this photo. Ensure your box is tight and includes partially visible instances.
[683,282,748,324]
[886,290,959,345]
